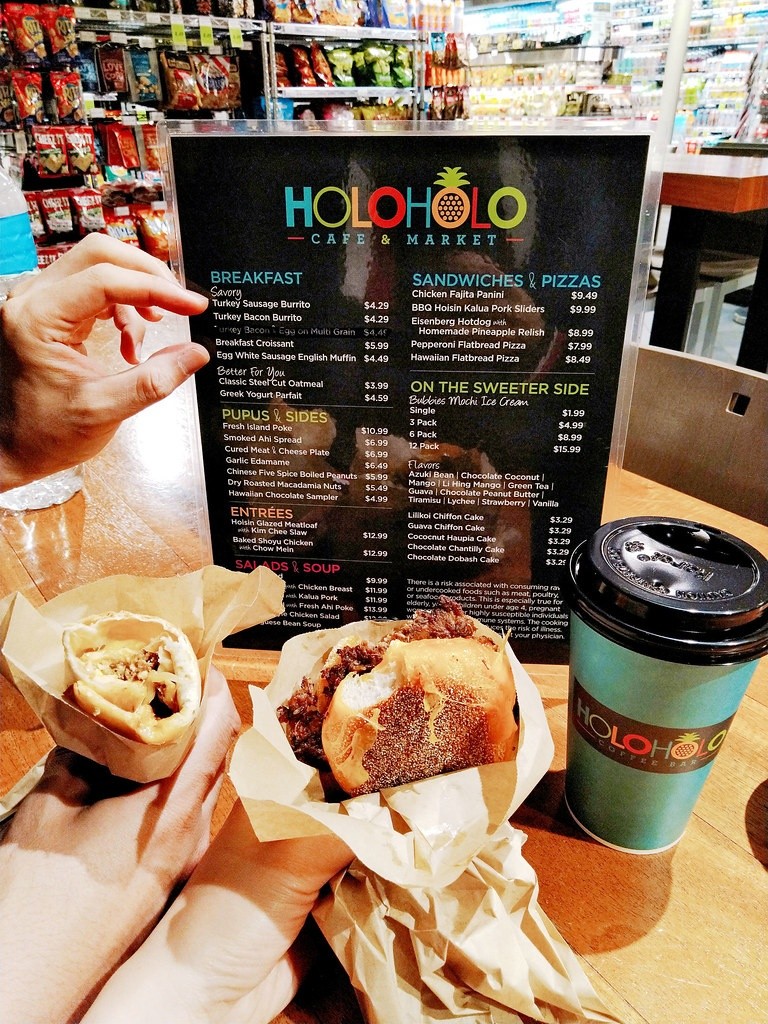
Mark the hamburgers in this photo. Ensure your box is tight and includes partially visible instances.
[276,594,518,798]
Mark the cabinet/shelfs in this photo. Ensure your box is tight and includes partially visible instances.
[0,0,768,271]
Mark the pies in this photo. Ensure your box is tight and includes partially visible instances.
[61,611,202,747]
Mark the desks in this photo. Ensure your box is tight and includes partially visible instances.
[647,152,768,416]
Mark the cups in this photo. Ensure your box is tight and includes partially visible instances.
[563,517,768,856]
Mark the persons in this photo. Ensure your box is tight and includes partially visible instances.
[79,797,356,1024]
[0,231,243,1024]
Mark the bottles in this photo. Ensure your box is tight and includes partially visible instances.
[0,165,39,304]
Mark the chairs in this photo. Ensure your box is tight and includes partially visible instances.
[618,344,768,530]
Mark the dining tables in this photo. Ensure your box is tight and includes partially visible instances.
[0,307,768,1024]
